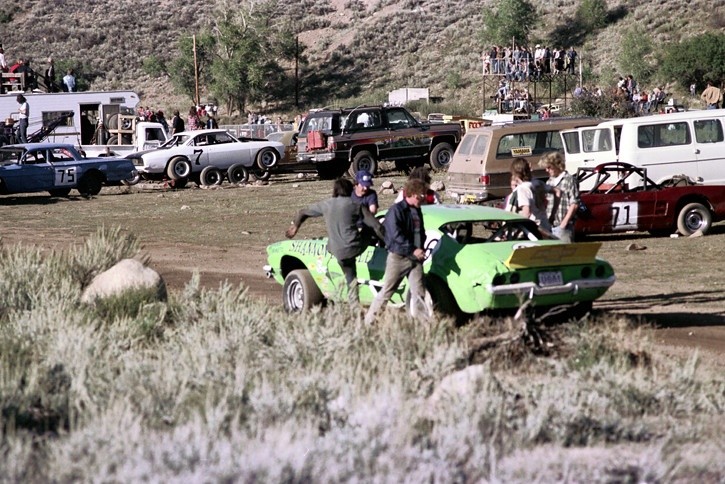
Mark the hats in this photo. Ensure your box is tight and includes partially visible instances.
[355,170,374,186]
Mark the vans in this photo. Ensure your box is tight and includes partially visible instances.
[558,108,725,193]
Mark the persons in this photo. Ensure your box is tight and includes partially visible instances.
[481,45,725,121]
[138,103,219,145]
[0,48,76,159]
[247,108,369,140]
[285,152,580,326]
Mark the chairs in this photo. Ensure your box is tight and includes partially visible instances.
[658,178,687,188]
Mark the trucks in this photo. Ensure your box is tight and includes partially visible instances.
[0,89,143,146]
[80,122,169,159]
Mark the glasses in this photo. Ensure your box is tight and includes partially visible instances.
[411,192,427,199]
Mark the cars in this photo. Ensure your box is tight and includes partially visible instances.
[481,162,725,243]
[0,142,139,197]
[257,130,320,176]
[126,128,286,185]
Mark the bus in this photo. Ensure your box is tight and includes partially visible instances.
[443,117,616,204]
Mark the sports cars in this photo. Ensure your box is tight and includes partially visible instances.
[263,203,615,330]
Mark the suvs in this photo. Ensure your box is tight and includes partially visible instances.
[292,104,462,181]
